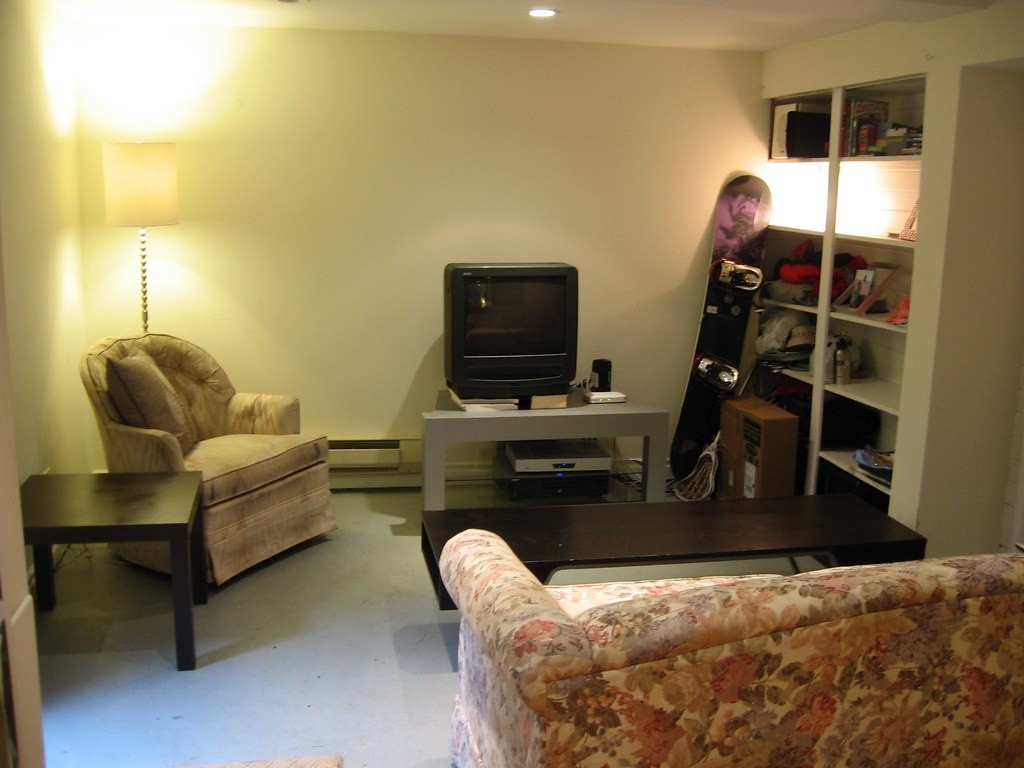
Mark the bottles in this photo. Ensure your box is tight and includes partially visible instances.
[835,342,851,384]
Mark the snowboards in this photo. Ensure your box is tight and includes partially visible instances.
[668,174,770,482]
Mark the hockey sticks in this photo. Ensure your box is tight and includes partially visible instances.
[672,356,758,504]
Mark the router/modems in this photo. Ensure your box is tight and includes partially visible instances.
[584,392,626,403]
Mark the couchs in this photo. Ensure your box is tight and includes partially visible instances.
[80,331,337,586]
[438,527,1024,768]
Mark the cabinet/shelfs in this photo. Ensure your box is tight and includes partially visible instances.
[750,76,926,516]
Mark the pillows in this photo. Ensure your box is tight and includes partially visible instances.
[102,343,197,457]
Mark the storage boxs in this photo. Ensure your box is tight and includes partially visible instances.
[771,103,830,159]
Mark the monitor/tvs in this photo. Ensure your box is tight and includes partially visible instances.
[444,260,578,398]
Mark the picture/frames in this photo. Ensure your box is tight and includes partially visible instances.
[899,197,917,243]
[832,261,903,316]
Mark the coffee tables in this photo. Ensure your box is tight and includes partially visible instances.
[420,493,928,609]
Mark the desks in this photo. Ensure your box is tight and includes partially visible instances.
[20,469,203,671]
[421,389,669,510]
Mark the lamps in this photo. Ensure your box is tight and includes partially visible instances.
[98,140,179,337]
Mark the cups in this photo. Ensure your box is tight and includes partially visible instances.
[592,359,611,392]
